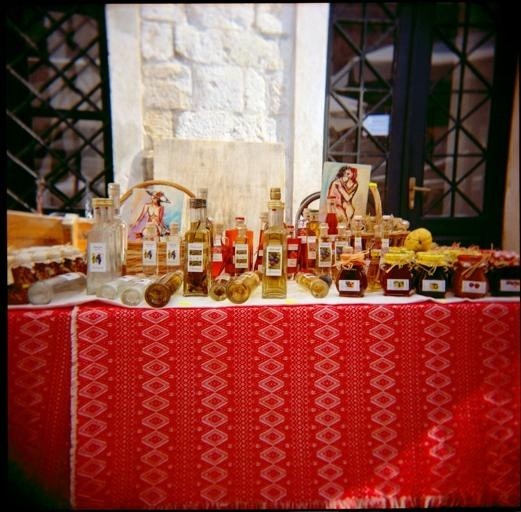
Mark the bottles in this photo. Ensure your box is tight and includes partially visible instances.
[84,178,391,307]
[28,267,83,306]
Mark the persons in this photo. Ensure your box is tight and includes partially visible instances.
[328,166,355,228]
[40,138,106,210]
[340,168,358,227]
[129,191,171,237]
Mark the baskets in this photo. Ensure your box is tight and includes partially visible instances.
[346,185,409,259]
[119,179,197,276]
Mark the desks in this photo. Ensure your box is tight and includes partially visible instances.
[7,282,519,512]
